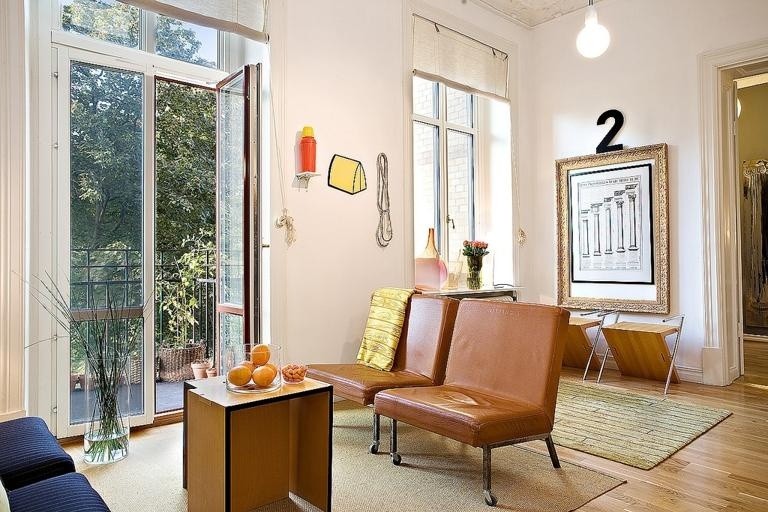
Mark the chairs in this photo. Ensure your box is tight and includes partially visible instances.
[373,293,570,505]
[305,291,460,454]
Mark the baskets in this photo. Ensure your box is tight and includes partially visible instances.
[158,342,205,382]
[125,353,158,386]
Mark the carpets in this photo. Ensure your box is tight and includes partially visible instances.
[77,408,628,511]
[543,372,733,473]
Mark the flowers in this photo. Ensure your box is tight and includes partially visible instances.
[462,238,491,286]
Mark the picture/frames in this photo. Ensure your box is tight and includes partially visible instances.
[553,142,672,317]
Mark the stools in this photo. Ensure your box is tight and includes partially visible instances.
[1,463,111,511]
[1,415,75,491]
[597,307,686,397]
[561,316,604,383]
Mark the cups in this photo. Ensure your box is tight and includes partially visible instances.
[444,261,463,289]
[225,344,283,394]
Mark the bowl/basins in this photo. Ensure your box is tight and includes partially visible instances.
[281,369,307,384]
[413,257,449,290]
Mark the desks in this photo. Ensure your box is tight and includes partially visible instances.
[186,375,336,512]
[435,288,516,305]
[180,374,234,489]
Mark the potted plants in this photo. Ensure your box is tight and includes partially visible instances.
[157,227,227,387]
[9,256,162,463]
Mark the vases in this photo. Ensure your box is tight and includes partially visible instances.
[467,265,485,291]
[208,367,218,379]
[189,361,208,376]
[414,228,447,293]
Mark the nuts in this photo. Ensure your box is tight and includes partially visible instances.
[282,364,306,383]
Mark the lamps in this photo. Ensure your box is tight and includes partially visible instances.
[577,1,611,58]
[297,125,320,191]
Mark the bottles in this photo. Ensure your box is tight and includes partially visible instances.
[300,127,315,171]
[419,228,440,291]
